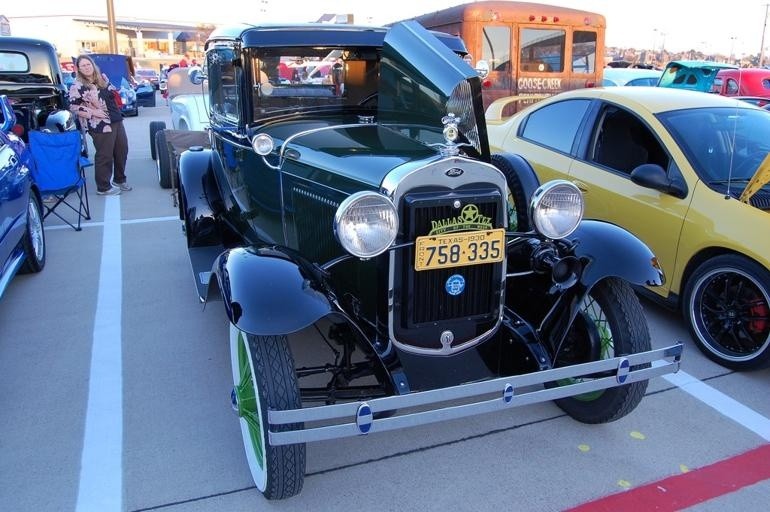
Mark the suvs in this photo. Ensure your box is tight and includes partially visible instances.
[0,36,70,142]
[0,94,46,302]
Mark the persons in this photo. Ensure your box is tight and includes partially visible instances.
[163,58,201,107]
[303,66,320,78]
[66,55,133,195]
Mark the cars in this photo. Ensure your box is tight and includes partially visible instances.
[605,60,770,113]
[61,53,156,117]
[170,21,685,501]
[463,86,770,372]
[150,66,210,189]
[135,69,160,90]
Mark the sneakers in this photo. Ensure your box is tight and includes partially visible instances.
[96,181,132,195]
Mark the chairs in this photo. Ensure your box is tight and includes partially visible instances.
[272,86,333,108]
[21,128,95,236]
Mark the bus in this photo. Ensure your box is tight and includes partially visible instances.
[396,0,607,117]
